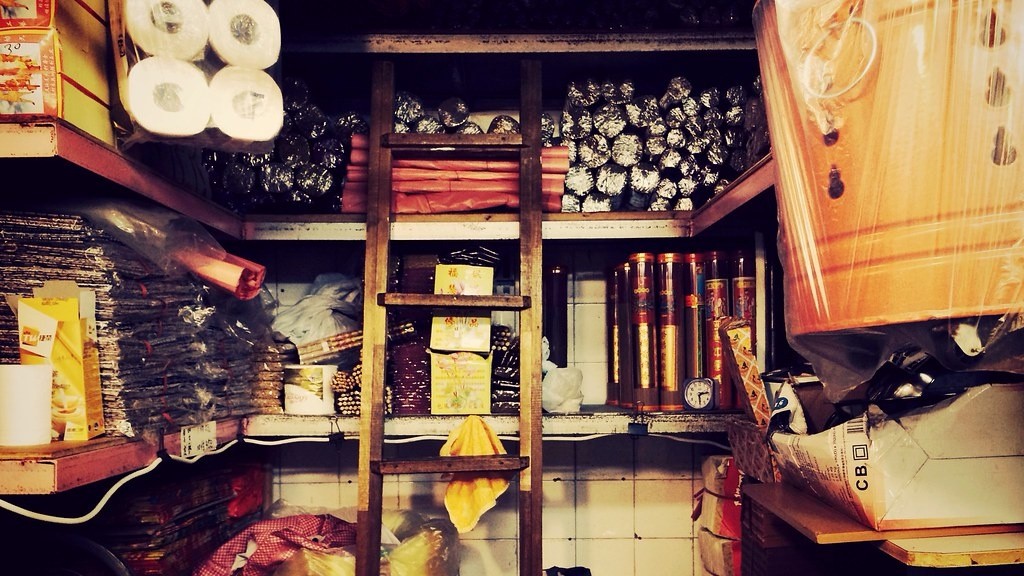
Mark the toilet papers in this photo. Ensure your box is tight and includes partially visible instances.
[120,0,286,147]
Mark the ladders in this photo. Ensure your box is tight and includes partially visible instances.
[354,50,547,575]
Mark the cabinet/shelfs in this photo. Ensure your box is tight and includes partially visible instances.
[0,0,1024,572]
